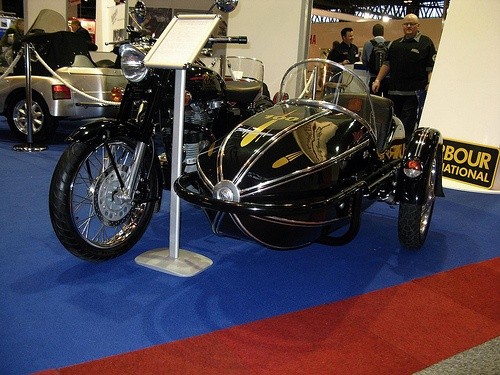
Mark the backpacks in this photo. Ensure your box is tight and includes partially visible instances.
[369,37,389,76]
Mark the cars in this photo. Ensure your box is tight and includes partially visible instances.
[0,9,126,143]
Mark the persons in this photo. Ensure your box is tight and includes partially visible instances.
[0,19,24,67]
[326,13,437,141]
[70,19,93,42]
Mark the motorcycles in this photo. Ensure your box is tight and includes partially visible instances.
[48,1,445,264]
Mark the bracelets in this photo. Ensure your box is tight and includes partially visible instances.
[372,79,381,84]
